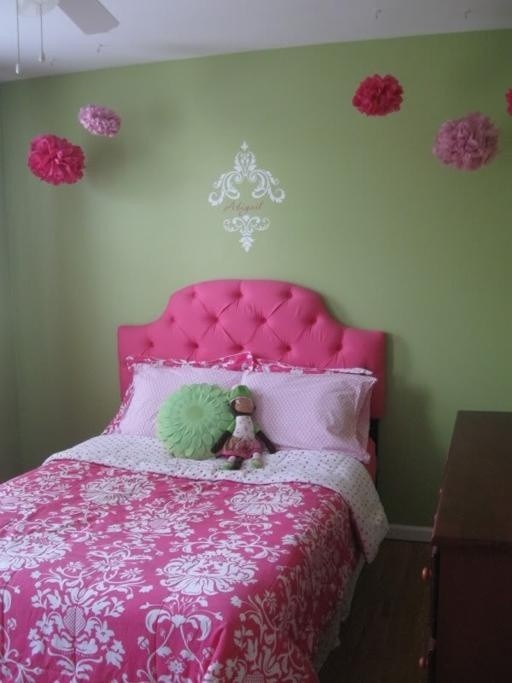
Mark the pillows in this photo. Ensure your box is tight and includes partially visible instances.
[242,354,377,463]
[102,350,253,438]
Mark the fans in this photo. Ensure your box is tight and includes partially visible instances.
[1,0,120,36]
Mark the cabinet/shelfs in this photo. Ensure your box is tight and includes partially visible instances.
[419,409,512,681]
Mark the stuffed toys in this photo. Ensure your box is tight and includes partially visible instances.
[211,383,278,471]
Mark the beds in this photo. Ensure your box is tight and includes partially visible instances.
[1,277,390,680]
